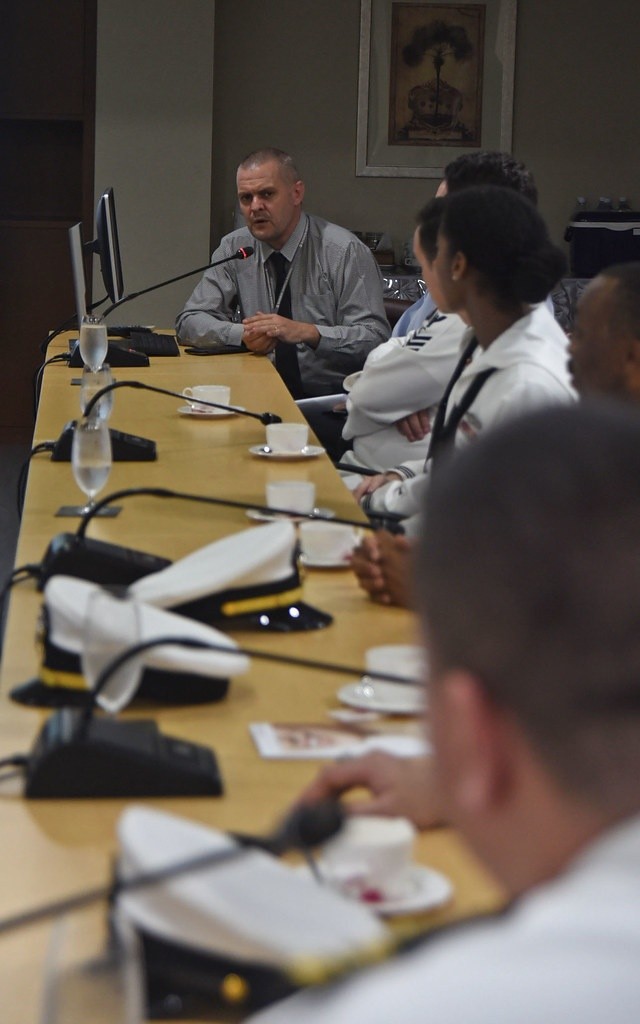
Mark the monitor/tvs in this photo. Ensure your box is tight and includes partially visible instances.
[70,186,150,369]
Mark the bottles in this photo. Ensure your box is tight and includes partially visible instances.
[575,196,630,211]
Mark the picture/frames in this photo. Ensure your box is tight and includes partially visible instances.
[355,0,521,179]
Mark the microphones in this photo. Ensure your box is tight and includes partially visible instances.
[36,487,406,594]
[49,382,282,461]
[0,798,347,930]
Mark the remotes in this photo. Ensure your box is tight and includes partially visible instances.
[105,326,150,336]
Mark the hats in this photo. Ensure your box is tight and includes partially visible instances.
[129,515,335,633]
[109,801,389,1024]
[7,577,252,709]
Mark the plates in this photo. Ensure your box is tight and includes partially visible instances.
[299,859,454,915]
[246,506,336,523]
[248,445,327,459]
[298,554,352,568]
[176,405,247,417]
[337,680,427,716]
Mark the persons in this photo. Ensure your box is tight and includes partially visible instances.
[334,197,468,492]
[298,749,449,830]
[355,186,582,539]
[174,147,392,400]
[295,152,537,464]
[348,261,640,608]
[242,392,640,1024]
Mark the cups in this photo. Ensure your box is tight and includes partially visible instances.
[79,362,115,423]
[265,479,315,516]
[182,384,231,412]
[296,519,353,561]
[342,814,415,899]
[365,643,428,704]
[79,587,141,714]
[366,231,383,250]
[77,313,109,374]
[265,423,308,454]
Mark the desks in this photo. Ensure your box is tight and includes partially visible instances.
[0,324,514,1024]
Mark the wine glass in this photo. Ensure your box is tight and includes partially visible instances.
[70,417,114,515]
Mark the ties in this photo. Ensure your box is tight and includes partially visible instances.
[268,252,305,400]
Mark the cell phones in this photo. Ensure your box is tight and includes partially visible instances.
[185,345,246,355]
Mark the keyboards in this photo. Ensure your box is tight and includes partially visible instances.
[131,331,180,358]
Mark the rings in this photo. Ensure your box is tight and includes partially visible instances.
[274,324,277,330]
